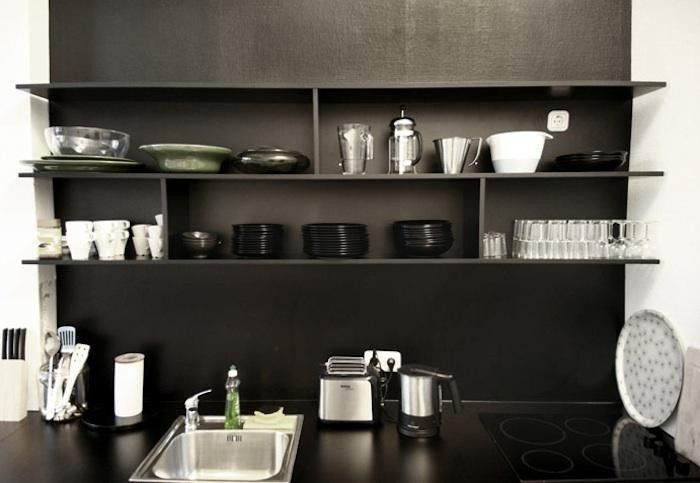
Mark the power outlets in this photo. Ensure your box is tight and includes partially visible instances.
[546,108,570,131]
[365,349,402,372]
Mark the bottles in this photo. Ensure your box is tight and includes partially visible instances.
[386,104,423,173]
[224,364,241,428]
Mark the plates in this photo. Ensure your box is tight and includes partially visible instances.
[393,219,455,255]
[614,307,684,428]
[301,222,372,256]
[230,221,285,257]
[554,149,629,168]
[19,155,143,171]
[610,417,672,479]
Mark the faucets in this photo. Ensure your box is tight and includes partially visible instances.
[182,387,214,431]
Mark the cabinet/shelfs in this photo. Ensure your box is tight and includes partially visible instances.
[15,79,670,264]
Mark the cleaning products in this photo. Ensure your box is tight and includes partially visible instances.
[222,363,243,430]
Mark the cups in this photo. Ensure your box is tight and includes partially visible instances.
[431,133,483,174]
[512,218,610,259]
[337,122,375,174]
[482,230,508,258]
[65,213,163,260]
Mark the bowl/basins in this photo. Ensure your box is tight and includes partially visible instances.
[179,227,222,258]
[484,130,555,173]
[43,124,132,157]
[138,140,232,171]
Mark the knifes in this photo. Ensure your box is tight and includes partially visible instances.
[2,326,26,359]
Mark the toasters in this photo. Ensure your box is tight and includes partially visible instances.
[318,356,382,424]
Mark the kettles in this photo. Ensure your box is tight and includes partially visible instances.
[396,363,462,438]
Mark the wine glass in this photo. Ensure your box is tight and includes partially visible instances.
[610,218,655,260]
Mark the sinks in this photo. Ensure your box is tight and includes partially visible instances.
[128,427,301,482]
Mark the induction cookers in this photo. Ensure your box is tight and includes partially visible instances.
[479,409,700,480]
